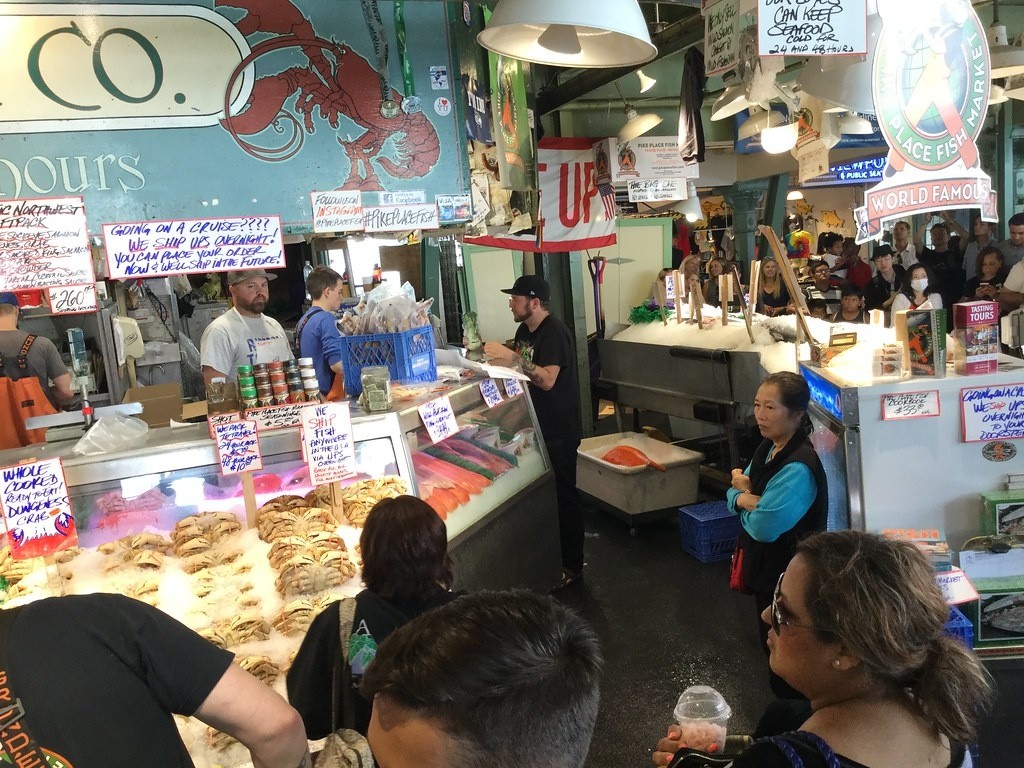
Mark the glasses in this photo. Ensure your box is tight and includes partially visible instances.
[818,269,830,272]
[771,573,832,637]
[763,256,776,260]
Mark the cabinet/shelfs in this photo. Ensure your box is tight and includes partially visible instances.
[18,298,131,412]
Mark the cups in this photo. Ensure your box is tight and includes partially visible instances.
[673,684,732,757]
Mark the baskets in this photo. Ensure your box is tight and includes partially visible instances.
[338,325,437,395]
[679,500,743,563]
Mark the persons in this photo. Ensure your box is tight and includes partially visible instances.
[484,275,583,592]
[0,292,74,450]
[726,371,829,704]
[997,256,1024,359]
[652,530,990,768]
[294,264,343,398]
[0,593,312,768]
[286,496,473,768]
[966,324,997,345]
[655,211,970,322]
[863,244,909,327]
[360,588,600,768]
[829,284,870,325]
[997,212,1024,271]
[962,214,999,282]
[199,268,329,411]
[956,247,1012,355]
[889,263,944,328]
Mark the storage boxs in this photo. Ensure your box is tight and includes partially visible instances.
[576,431,706,515]
[896,300,998,379]
[938,489,1024,768]
[338,325,438,396]
[122,383,208,428]
[679,501,745,564]
[146,277,172,295]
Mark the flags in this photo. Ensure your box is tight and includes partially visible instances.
[597,183,616,222]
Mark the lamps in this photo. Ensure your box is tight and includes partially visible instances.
[613,69,875,154]
[671,183,703,222]
[988,0,1024,105]
[476,0,658,68]
[787,179,803,200]
[694,203,734,243]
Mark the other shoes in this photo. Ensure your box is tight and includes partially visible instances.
[552,569,581,591]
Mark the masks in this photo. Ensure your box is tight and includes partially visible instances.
[911,279,928,291]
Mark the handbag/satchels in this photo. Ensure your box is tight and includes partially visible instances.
[314,729,374,768]
[729,536,745,592]
[668,748,737,768]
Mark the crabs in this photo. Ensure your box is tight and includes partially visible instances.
[0,471,407,768]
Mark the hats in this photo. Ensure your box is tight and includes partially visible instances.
[869,244,897,261]
[227,270,277,287]
[0,292,25,318]
[501,275,551,298]
